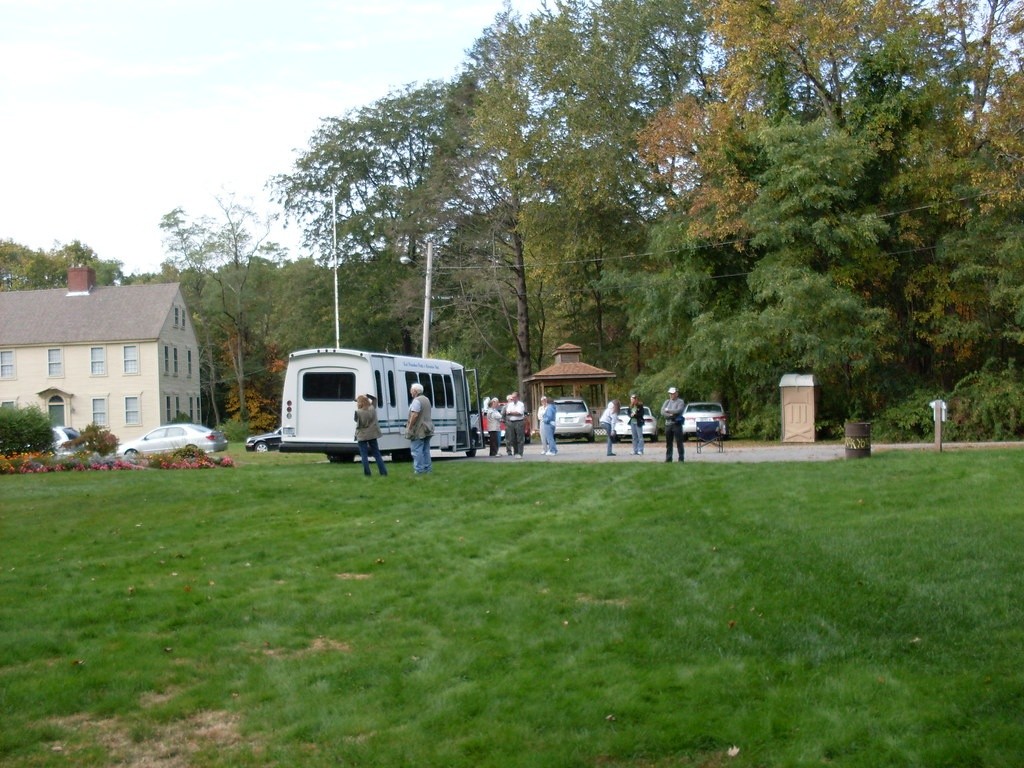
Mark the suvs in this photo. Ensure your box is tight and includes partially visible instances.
[477,402,531,444]
[552,398,597,442]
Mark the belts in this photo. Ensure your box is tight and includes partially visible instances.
[510,419,523,422]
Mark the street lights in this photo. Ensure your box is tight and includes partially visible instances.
[399,241,435,358]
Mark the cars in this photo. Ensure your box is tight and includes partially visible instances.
[681,402,727,441]
[610,406,659,443]
[25,426,84,455]
[244,427,281,453]
[111,422,229,459]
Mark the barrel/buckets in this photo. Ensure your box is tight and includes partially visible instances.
[843,422,871,459]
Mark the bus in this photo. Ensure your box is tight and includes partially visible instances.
[276,347,485,464]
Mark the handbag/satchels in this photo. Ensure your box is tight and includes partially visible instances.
[674,414,685,425]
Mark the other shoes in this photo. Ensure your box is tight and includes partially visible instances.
[546,449,559,456]
[630,450,637,455]
[637,451,643,455]
[541,450,547,455]
[607,453,616,456]
[495,454,502,457]
[508,451,512,455]
[514,453,523,459]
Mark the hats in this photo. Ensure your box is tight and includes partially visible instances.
[667,387,677,394]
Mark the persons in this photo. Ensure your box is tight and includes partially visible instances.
[661,387,685,463]
[487,398,503,456]
[404,383,435,475]
[600,398,621,456]
[627,394,646,456]
[501,393,524,459]
[537,396,558,455]
[354,394,388,476]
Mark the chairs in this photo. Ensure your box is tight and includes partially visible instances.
[697,420,724,455]
